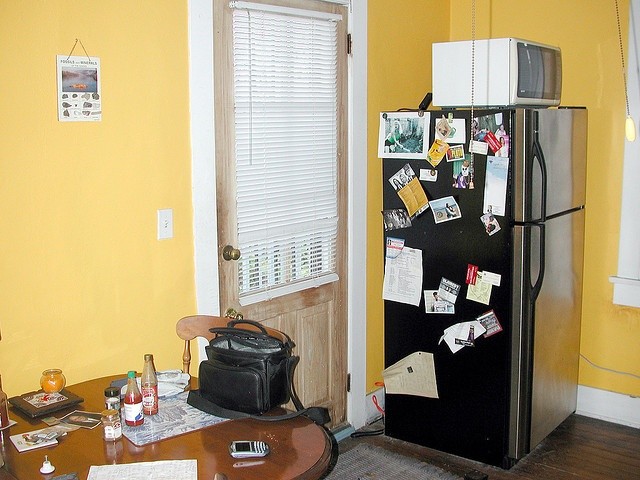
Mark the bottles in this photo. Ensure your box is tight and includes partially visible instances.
[104,386,121,421]
[124,371,144,427]
[0,374,10,428]
[141,354,159,415]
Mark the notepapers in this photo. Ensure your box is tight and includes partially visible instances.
[420,169,438,182]
[465,270,493,305]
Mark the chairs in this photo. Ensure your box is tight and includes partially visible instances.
[176,315,291,374]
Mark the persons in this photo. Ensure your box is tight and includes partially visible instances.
[449,207,458,217]
[445,202,454,219]
[482,216,495,226]
[69,413,101,428]
[392,179,402,189]
[495,124,508,137]
[399,174,408,187]
[487,223,495,234]
[498,138,507,156]
[432,293,441,311]
[439,129,448,136]
[404,169,416,182]
[22,434,41,445]
[472,120,483,137]
[384,121,406,153]
[397,210,407,228]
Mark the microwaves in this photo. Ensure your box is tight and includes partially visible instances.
[432,37,562,109]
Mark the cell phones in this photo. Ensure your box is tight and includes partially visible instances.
[226,440,271,459]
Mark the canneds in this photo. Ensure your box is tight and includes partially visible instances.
[106,387,120,414]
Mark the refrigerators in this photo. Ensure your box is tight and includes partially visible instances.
[382,106,589,470]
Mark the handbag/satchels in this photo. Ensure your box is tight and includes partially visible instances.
[186,319,332,428]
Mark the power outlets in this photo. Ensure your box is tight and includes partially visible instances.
[156,208,174,239]
